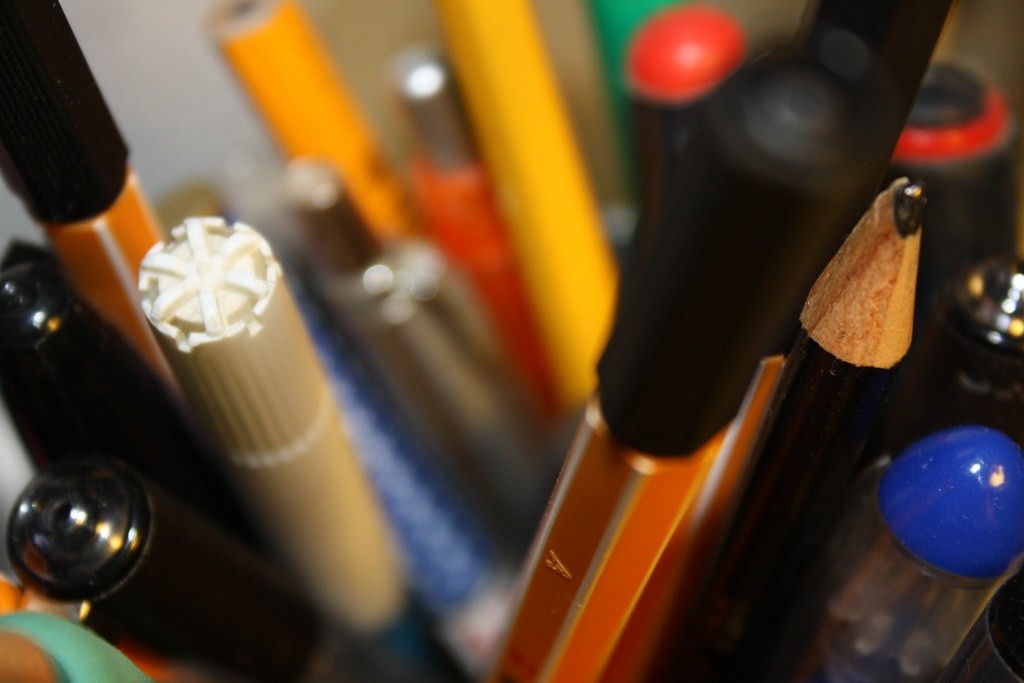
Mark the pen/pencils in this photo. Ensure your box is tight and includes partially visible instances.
[428,1,623,423]
[202,1,416,256]
[669,174,927,683]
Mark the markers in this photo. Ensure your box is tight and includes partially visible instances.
[0,0,1024,683]
